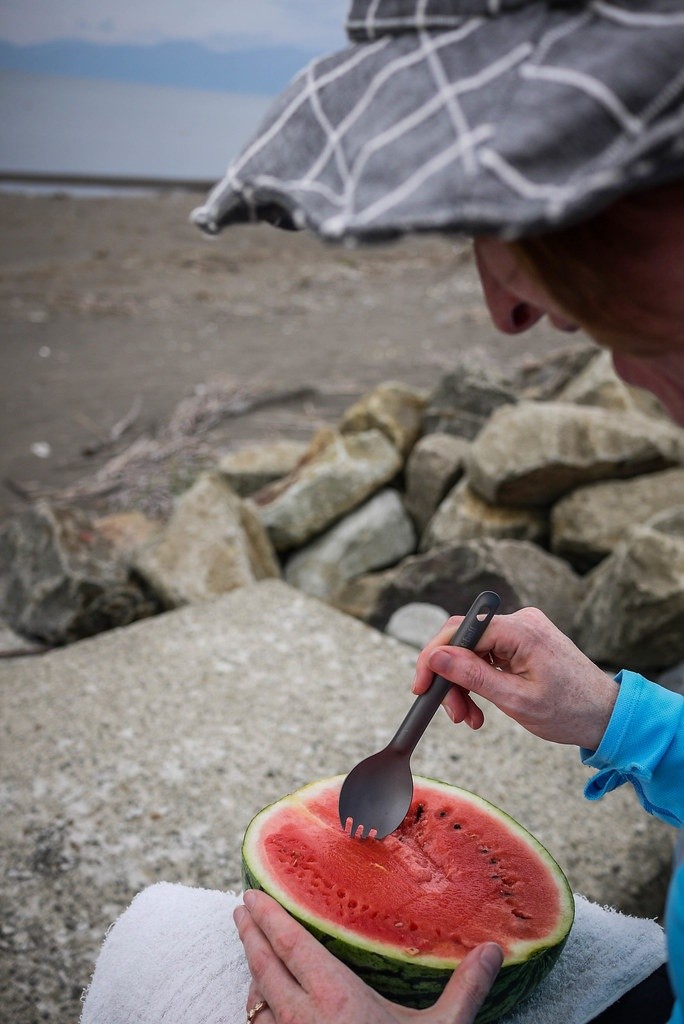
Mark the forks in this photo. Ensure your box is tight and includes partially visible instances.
[338,590,502,840]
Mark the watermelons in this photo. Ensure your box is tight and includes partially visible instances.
[239,772,576,1024]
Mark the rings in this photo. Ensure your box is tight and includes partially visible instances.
[247,1001,267,1024]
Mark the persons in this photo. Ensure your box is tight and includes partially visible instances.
[192,0,684,1024]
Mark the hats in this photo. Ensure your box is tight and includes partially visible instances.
[190,0,684,248]
